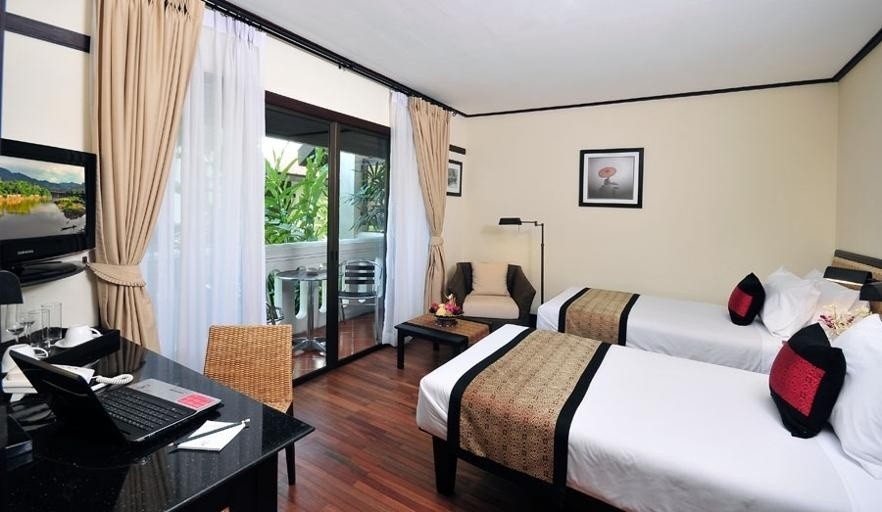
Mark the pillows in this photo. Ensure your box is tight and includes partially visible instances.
[827,311,882,477]
[823,264,871,294]
[799,270,858,331]
[768,325,844,440]
[758,265,819,341]
[471,262,509,297]
[727,272,767,327]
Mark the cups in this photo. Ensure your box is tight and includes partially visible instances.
[27,309,51,351]
[2,343,49,374]
[64,324,102,346]
[40,301,62,342]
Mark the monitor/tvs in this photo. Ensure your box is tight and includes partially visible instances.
[0,137,96,287]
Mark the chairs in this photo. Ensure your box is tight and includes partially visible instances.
[448,262,536,333]
[265,302,285,325]
[337,259,385,347]
[202,324,304,482]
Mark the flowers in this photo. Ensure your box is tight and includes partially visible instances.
[819,303,876,337]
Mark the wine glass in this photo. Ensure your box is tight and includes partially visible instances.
[5,293,37,347]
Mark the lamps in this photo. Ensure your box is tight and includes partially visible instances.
[497,217,544,306]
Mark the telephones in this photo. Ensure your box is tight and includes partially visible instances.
[1,361,95,394]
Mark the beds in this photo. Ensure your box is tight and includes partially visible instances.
[535,249,882,375]
[413,325,882,512]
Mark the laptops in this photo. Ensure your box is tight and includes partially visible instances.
[9,349,224,449]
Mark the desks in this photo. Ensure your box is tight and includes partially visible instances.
[0,327,314,512]
[393,309,492,370]
[276,267,342,356]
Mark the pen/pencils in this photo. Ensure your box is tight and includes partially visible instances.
[167,418,251,448]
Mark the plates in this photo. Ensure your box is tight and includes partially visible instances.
[55,339,73,348]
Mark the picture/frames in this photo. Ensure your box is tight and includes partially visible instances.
[447,159,462,196]
[579,147,644,209]
